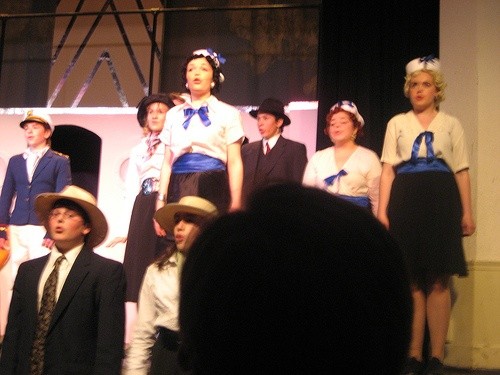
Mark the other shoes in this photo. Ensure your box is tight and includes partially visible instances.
[424,358,443,375]
[405,357,421,375]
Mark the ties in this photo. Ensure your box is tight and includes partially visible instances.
[29,255,66,375]
[266,142,270,153]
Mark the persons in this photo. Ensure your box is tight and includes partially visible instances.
[0,109,72,304]
[303,100,383,220]
[154,47,245,261]
[0,185,124,375]
[377,58,475,371]
[107,94,176,357]
[240,98,309,210]
[179,182,414,375]
[126,196,218,375]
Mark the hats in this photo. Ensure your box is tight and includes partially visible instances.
[249,98,291,125]
[405,48,440,76]
[19,108,55,134]
[155,196,217,234]
[329,100,365,128]
[136,94,176,127]
[34,184,108,249]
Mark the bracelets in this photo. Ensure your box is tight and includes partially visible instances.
[157,194,166,202]
